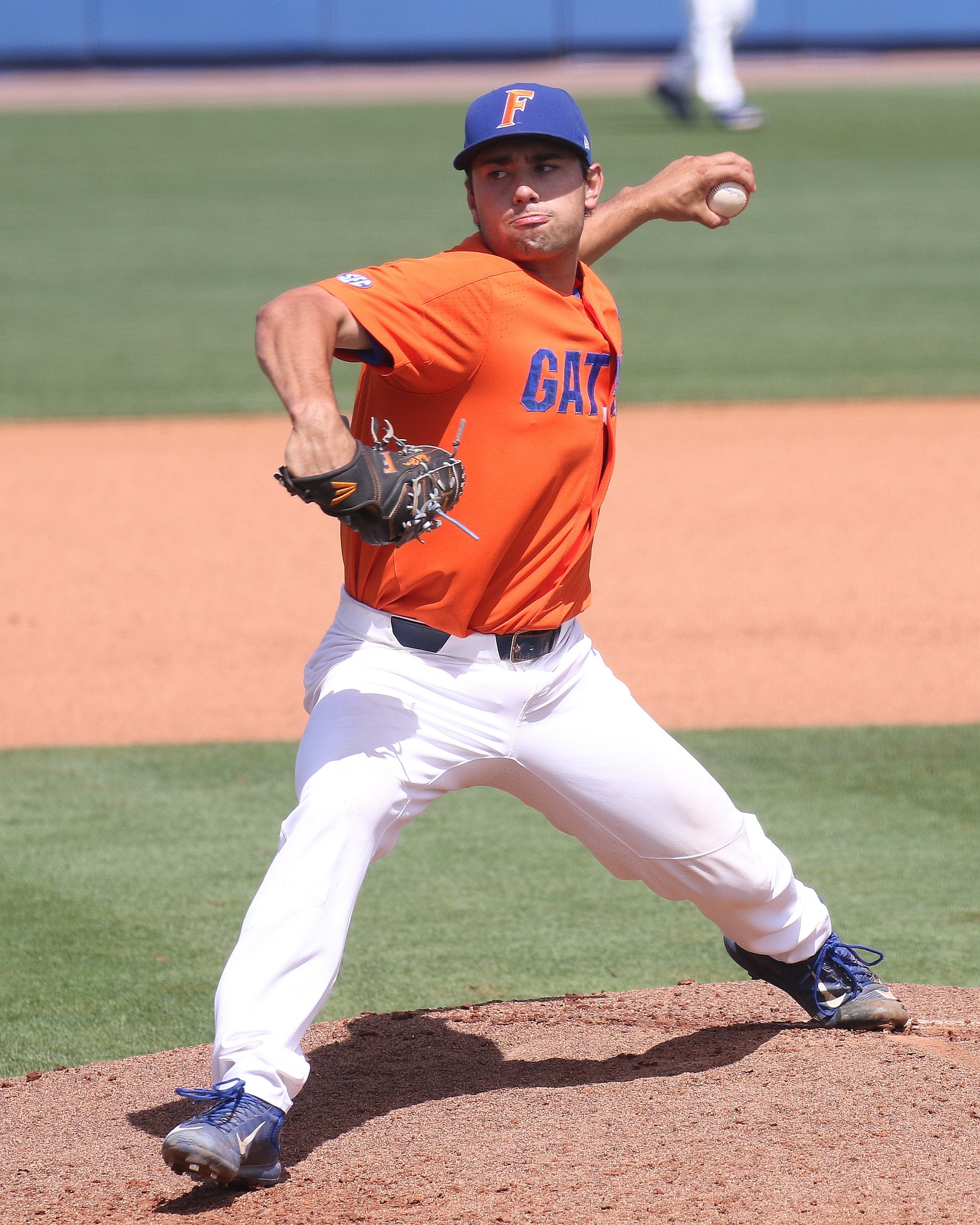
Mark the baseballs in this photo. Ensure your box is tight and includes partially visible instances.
[708,180,750,217]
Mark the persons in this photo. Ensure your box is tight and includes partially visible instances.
[652,0,766,131]
[161,82,910,1184]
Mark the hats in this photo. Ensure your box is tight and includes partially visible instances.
[453,83,592,170]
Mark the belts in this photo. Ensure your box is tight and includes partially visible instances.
[391,616,561,664]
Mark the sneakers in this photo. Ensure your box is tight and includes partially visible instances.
[724,931,908,1025]
[163,1078,284,1183]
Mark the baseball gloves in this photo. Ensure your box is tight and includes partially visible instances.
[274,415,468,540]
